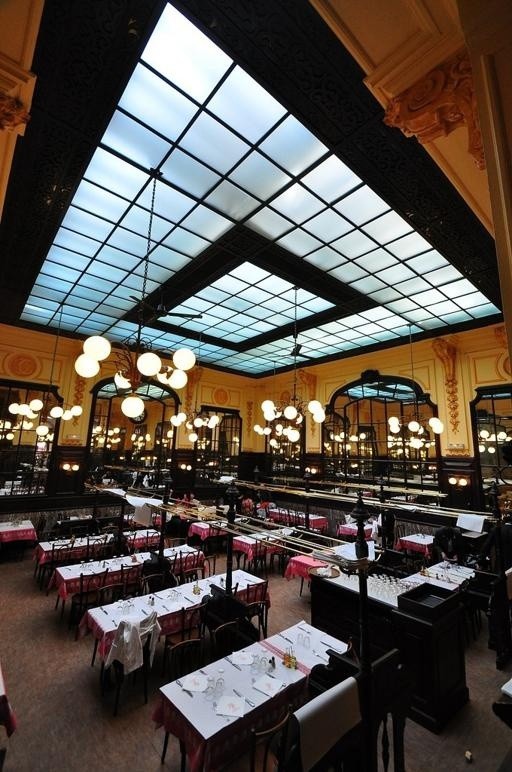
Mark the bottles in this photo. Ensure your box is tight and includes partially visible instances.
[284,646,297,670]
[206,668,225,692]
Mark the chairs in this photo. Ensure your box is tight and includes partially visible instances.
[34,487,435,716]
[246,703,300,771]
[282,676,390,771]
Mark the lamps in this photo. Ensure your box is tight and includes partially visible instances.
[261,287,326,442]
[388,324,444,451]
[73,167,196,419]
[7,301,83,437]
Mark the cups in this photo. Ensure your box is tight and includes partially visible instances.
[296,631,310,648]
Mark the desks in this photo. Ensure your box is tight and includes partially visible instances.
[2,519,35,553]
[154,620,349,772]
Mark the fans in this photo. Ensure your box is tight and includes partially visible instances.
[129,285,203,324]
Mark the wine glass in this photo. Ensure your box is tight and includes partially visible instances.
[366,573,416,605]
[250,650,276,673]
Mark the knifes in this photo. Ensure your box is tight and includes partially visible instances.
[224,656,243,671]
[276,631,293,644]
[265,671,286,687]
[51,523,229,628]
[234,687,256,708]
[175,679,194,698]
[320,641,338,652]
